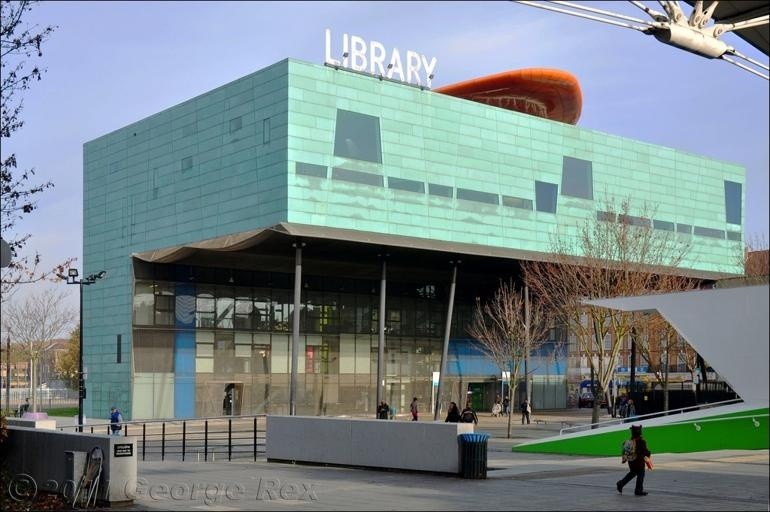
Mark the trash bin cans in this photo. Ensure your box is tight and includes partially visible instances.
[460,433,490,480]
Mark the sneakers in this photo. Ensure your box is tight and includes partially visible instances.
[616,481,623,493]
[634,490,648,495]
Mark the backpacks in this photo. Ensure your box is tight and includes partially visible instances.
[623,440,637,462]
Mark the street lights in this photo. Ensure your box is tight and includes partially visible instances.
[57,267,106,431]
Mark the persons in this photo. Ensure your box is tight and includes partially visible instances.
[618,394,627,415]
[616,424,651,496]
[460,402,478,425]
[20,398,32,417]
[520,399,532,424]
[491,394,511,417]
[620,399,636,422]
[378,400,390,419]
[111,406,123,434]
[445,402,465,422]
[410,397,418,421]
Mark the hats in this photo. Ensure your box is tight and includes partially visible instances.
[629,424,641,429]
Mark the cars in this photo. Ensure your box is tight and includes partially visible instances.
[581,393,607,408]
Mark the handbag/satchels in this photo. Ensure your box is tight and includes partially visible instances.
[644,456,655,471]
[527,404,531,414]
